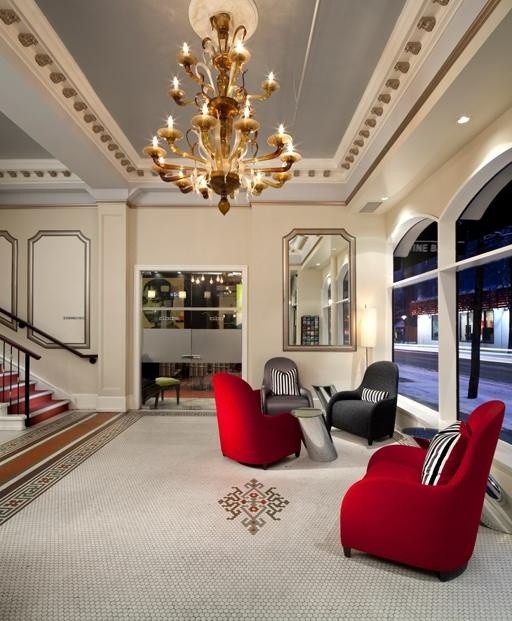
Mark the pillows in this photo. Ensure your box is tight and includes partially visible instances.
[422,420,470,486]
[270,368,300,396]
[362,387,388,403]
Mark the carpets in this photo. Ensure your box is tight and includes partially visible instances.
[0,409,512,620]
[142,397,216,410]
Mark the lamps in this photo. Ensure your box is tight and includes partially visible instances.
[143,1,302,216]
[190,275,234,300]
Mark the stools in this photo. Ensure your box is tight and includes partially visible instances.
[155,378,180,404]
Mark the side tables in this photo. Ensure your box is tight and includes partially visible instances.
[400,426,512,534]
[311,383,338,409]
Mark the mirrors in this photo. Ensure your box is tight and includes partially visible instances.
[141,271,242,329]
[282,228,357,352]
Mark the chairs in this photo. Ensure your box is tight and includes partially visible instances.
[326,361,399,446]
[341,401,506,582]
[213,372,302,470]
[260,357,313,415]
